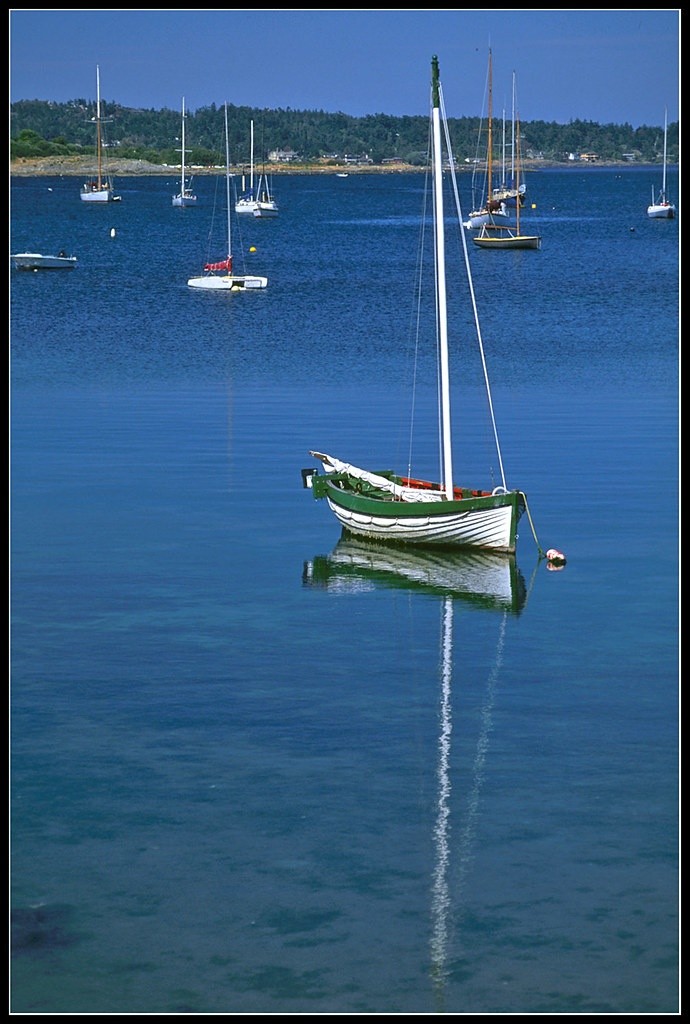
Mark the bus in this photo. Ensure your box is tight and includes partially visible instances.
[623,154,635,161]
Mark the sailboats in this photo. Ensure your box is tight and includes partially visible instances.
[298,522,528,1000]
[187,101,268,291]
[80,64,116,202]
[648,105,677,218]
[485,68,527,209]
[471,46,542,252]
[463,43,493,229]
[300,52,527,564]
[238,121,278,217]
[172,95,197,207]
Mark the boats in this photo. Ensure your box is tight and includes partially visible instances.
[11,247,77,272]
[337,173,349,178]
